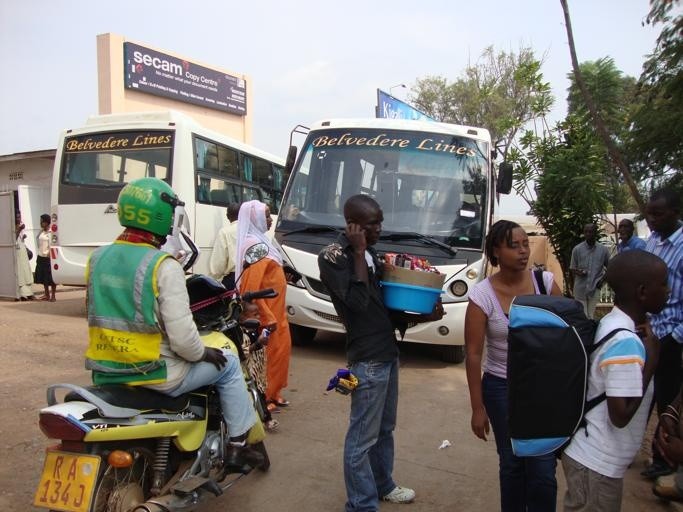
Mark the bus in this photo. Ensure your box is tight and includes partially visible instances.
[253,112,516,369]
[42,111,341,312]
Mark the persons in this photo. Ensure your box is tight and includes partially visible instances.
[617,219,646,252]
[15,210,37,301]
[569,224,611,319]
[318,196,446,512]
[34,214,58,301]
[235,200,292,412]
[562,249,671,512]
[466,220,564,512]
[84,177,267,469]
[211,200,235,292]
[643,188,683,479]
[652,388,683,499]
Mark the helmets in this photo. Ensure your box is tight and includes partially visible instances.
[117,178,186,238]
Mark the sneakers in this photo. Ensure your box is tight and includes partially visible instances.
[381,486,416,503]
[264,420,279,431]
[639,465,672,477]
[275,398,290,407]
[266,402,279,413]
[227,445,264,466]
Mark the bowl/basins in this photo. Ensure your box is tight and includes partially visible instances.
[380,262,447,314]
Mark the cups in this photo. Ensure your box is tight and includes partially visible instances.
[261,328,270,338]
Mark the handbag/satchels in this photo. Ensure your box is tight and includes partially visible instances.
[26,246,34,260]
[508,295,598,457]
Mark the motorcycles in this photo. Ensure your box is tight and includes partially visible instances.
[38,263,273,512]
